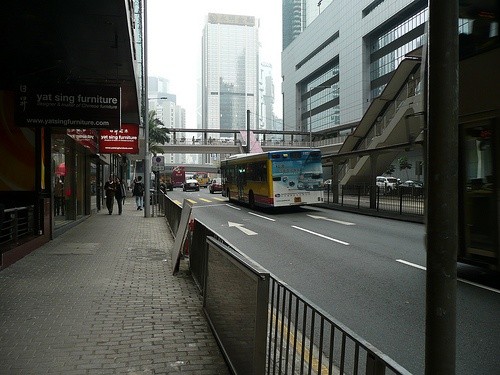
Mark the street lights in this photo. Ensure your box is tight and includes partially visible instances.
[310,86,331,149]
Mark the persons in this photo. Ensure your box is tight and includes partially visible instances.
[104,178,115,215]
[115,177,126,215]
[132,176,145,211]
[54,175,64,217]
[133,177,137,183]
[192,136,195,144]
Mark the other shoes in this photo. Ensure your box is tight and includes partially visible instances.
[137,206,142,210]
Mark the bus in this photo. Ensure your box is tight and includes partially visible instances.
[185,172,208,188]
[217,148,324,210]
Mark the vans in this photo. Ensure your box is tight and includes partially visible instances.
[375,176,400,194]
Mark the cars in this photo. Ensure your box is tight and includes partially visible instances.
[398,180,424,194]
[209,178,222,194]
[182,179,199,191]
